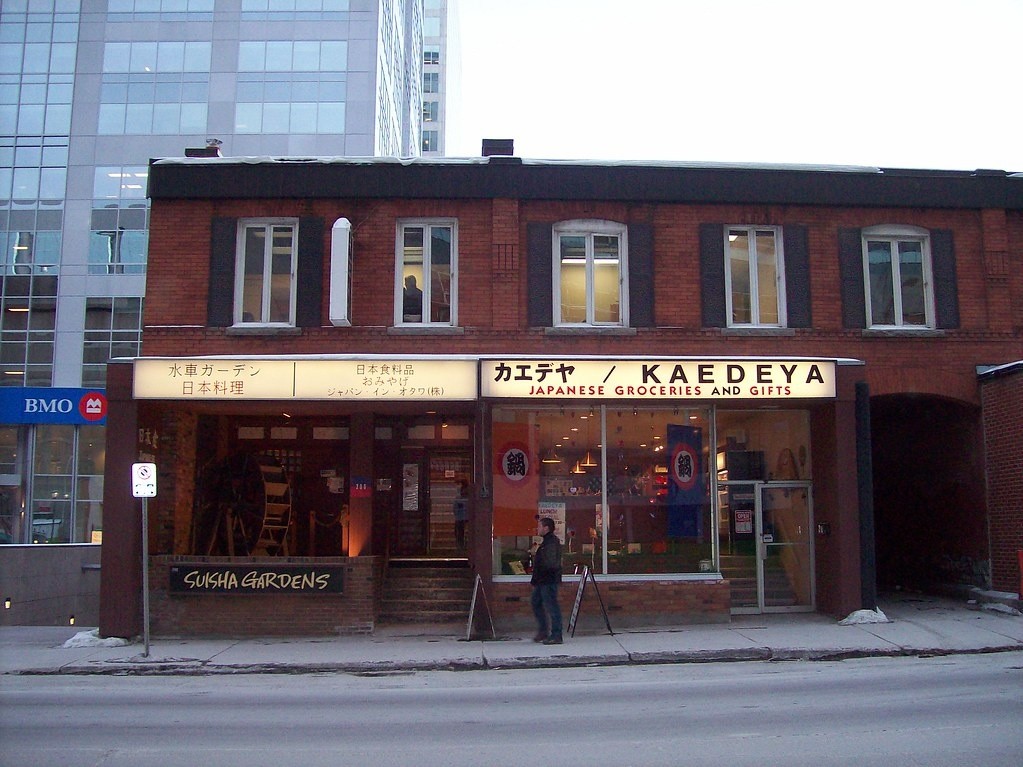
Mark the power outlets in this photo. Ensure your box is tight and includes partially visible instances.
[573,432,586,473]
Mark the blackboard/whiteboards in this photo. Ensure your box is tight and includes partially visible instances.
[569,566,589,628]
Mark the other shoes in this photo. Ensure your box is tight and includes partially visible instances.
[534,634,548,642]
[543,636,563,644]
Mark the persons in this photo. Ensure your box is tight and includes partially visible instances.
[404,276,422,322]
[452,479,470,551]
[531,517,563,644]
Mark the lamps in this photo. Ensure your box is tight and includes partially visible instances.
[580,420,599,467]
[633,407,639,416]
[673,407,679,415]
[542,414,561,463]
[559,405,565,415]
[51,491,59,541]
[589,406,594,416]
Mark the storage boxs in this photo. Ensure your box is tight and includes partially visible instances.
[628,543,641,554]
[90,531,102,543]
[582,544,594,554]
[33,519,62,540]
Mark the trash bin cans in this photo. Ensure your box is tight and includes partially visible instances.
[752,527,774,555]
